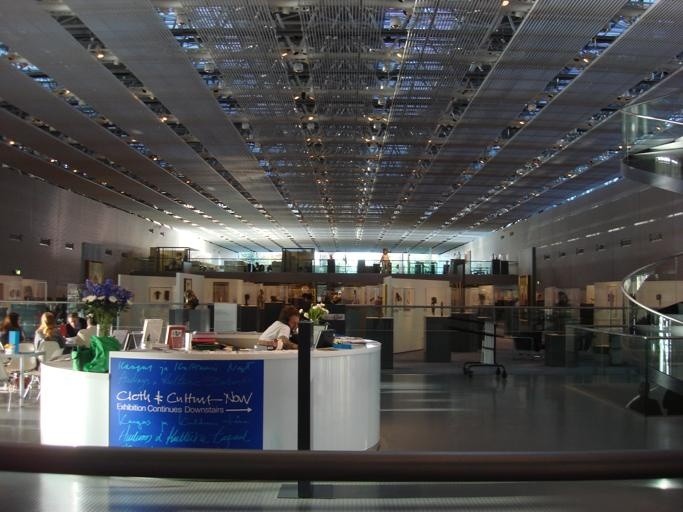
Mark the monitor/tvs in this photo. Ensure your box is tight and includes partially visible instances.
[316,329,335,348]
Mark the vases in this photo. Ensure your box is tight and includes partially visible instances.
[94,322,111,336]
[301,320,328,344]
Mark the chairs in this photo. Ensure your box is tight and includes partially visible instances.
[512,331,544,360]
[0,329,130,412]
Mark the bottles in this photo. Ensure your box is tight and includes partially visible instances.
[9,330,20,354]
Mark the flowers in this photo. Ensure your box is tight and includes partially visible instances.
[78,279,132,337]
[298,303,329,324]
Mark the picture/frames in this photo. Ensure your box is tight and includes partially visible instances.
[183,278,192,292]
[392,287,414,310]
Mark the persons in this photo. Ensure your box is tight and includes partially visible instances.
[254,304,299,350]
[0,312,25,348]
[34,311,69,356]
[63,313,85,338]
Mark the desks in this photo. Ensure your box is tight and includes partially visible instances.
[545,329,596,367]
